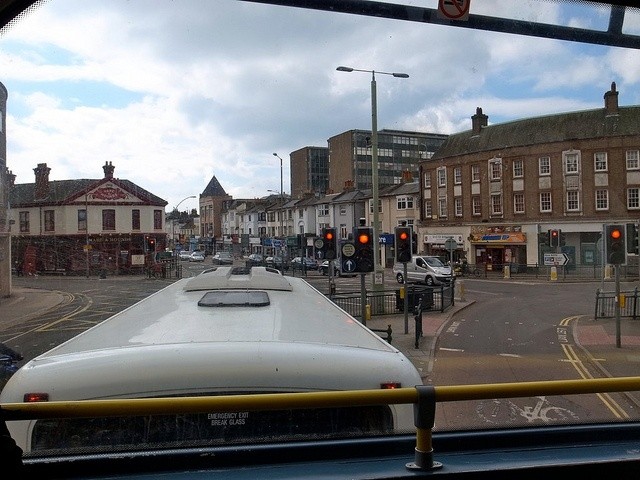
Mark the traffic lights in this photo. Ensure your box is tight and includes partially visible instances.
[148,239,155,252]
[604,223,628,266]
[322,228,337,259]
[626,223,636,253]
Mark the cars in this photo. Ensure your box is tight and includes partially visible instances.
[319,260,357,277]
[291,257,318,270]
[189,253,204,262]
[248,254,265,268]
[212,255,233,265]
[179,251,190,261]
[265,257,289,271]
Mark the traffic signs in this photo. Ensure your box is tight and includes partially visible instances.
[241,234,249,247]
[315,249,324,259]
[232,234,239,243]
[544,253,569,266]
[313,238,324,249]
[340,241,356,258]
[342,259,357,273]
[445,239,457,252]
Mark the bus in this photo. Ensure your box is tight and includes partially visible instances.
[0,266,435,453]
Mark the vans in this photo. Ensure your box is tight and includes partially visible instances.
[393,256,456,286]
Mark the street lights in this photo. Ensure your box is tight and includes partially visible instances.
[273,153,282,195]
[395,226,412,263]
[352,227,375,273]
[267,190,284,275]
[336,67,408,313]
[548,229,561,247]
[173,196,196,244]
